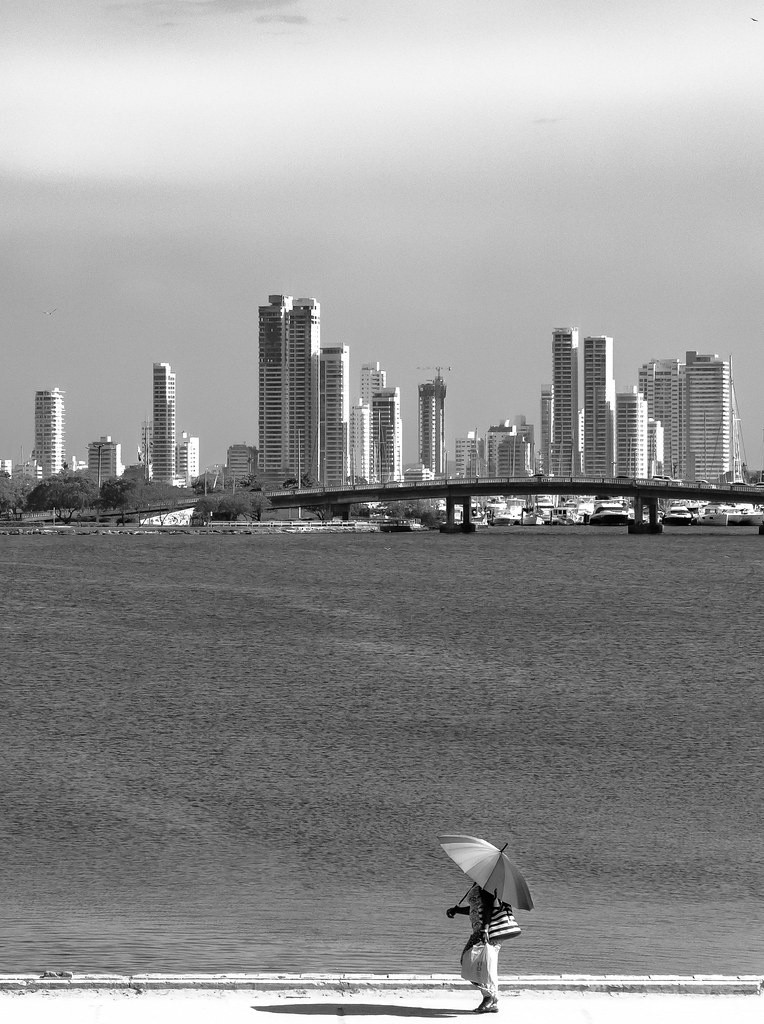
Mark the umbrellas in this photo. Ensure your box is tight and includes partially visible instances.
[437,834,535,918]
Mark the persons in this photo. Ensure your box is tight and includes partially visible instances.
[446,886,504,1013]
[698,481,704,488]
[476,473,479,483]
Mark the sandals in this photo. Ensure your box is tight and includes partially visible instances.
[474,996,499,1013]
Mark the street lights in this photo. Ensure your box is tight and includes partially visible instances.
[443,450,450,484]
[611,460,614,482]
[94,444,104,522]
[230,469,236,520]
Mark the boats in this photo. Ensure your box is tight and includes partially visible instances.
[380,518,413,533]
[439,495,665,526]
[663,500,763,526]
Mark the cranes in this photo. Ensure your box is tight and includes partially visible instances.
[416,366,451,380]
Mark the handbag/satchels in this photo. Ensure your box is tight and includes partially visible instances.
[478,886,521,945]
[460,940,501,987]
